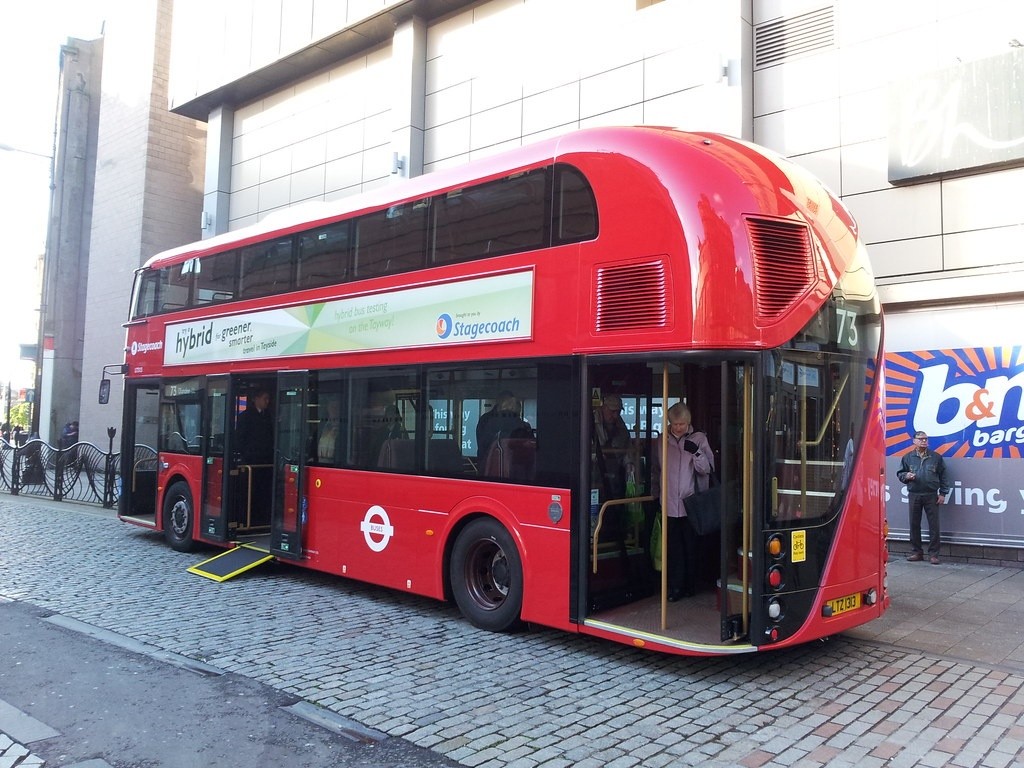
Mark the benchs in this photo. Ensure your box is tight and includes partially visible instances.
[376,438,463,475]
[483,437,538,483]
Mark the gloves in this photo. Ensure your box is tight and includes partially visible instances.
[683,440,699,455]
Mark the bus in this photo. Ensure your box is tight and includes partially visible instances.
[97,125,891,659]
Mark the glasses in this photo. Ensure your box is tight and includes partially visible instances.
[914,438,928,441]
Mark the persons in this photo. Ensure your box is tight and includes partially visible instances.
[897,431,950,564]
[842,423,855,489]
[591,394,632,482]
[234,383,409,528]
[476,391,535,476]
[650,401,715,603]
[63,422,79,465]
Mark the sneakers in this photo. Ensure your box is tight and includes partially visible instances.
[907,554,925,561]
[928,556,940,564]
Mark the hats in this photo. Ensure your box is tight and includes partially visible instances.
[496,394,522,416]
[603,392,624,411]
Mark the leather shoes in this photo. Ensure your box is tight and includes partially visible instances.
[667,585,695,601]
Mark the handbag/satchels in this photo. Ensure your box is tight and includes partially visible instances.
[682,462,742,536]
[648,507,663,571]
[623,472,646,528]
